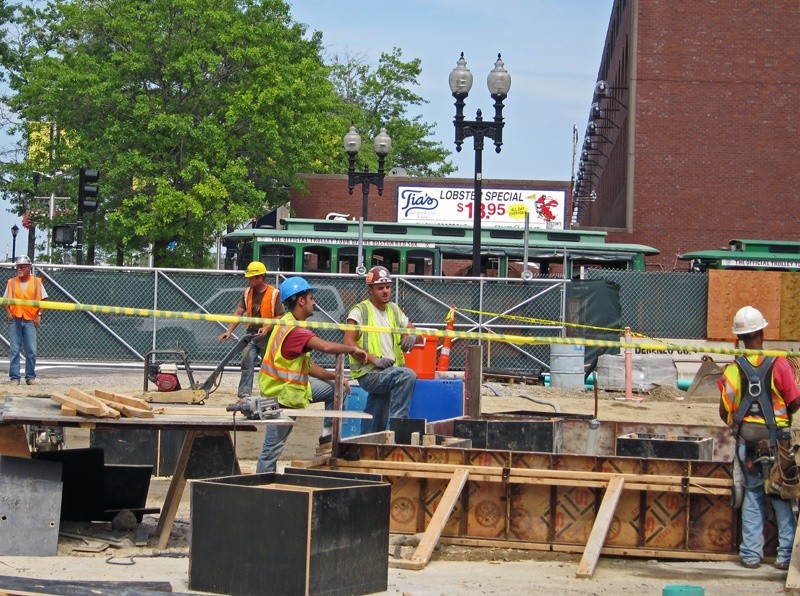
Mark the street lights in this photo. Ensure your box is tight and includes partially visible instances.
[448,52,512,330]
[11,224,18,269]
[342,124,392,220]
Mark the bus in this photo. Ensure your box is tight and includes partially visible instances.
[220,215,665,389]
[669,238,800,273]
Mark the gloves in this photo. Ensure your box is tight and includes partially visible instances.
[374,358,395,369]
[397,334,417,352]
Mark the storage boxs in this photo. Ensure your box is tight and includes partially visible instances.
[617,432,714,461]
[189,471,392,596]
[453,415,564,453]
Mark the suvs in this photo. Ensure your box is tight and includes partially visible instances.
[137,285,348,367]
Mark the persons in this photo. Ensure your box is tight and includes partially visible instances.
[343,266,422,435]
[217,261,285,404]
[716,306,800,568]
[3,254,48,385]
[256,276,367,473]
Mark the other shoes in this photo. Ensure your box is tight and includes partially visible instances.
[319,434,332,454]
[776,562,790,569]
[741,561,760,568]
[10,379,19,385]
[27,379,37,385]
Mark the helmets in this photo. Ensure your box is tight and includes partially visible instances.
[732,305,768,335]
[245,261,266,277]
[16,255,32,264]
[280,277,318,303]
[366,266,393,284]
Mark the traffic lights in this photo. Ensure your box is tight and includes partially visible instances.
[78,167,99,214]
[53,224,75,245]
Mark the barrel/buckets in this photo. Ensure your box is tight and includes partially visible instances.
[401,333,440,379]
[550,345,584,387]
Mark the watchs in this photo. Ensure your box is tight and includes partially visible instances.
[37,312,42,316]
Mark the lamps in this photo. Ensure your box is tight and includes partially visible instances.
[571,80,629,216]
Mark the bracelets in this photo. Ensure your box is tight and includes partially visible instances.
[349,347,359,356]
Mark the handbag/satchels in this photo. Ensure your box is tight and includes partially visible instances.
[767,438,800,501]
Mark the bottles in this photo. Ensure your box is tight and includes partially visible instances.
[789,444,800,455]
[750,404,760,415]
